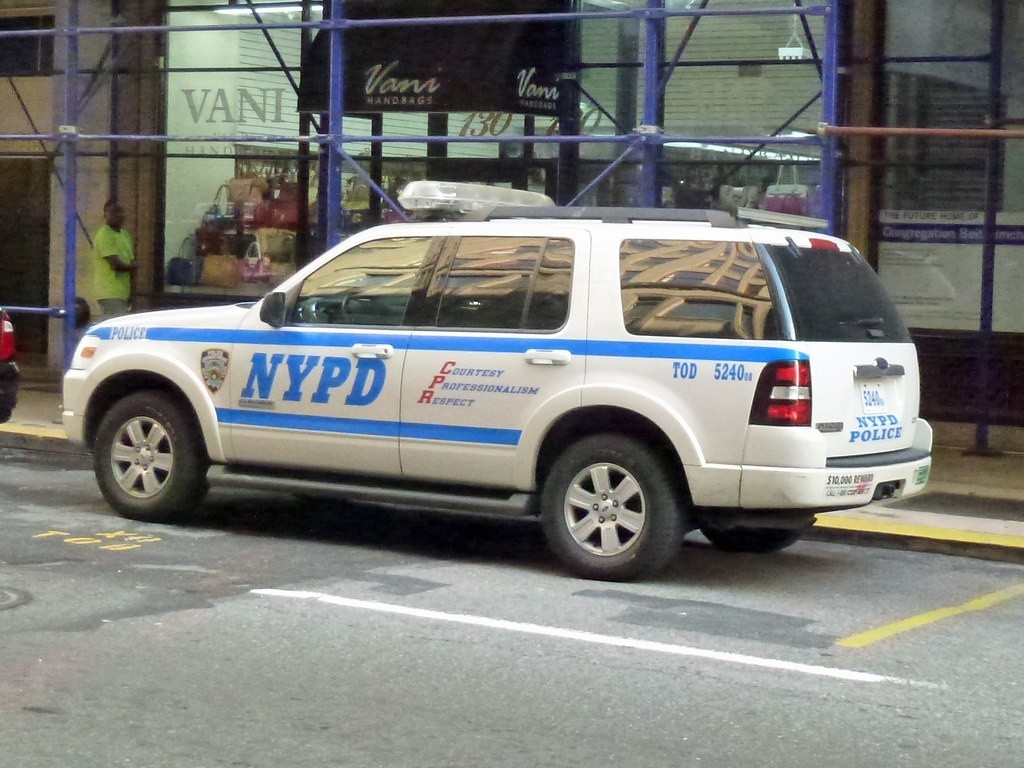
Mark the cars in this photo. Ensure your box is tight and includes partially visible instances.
[0,306,22,428]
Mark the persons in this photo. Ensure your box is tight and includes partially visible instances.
[94,199,138,319]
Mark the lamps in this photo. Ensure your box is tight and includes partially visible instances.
[778,3,822,62]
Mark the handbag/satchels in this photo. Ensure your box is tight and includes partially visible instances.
[674,162,822,217]
[164,162,300,286]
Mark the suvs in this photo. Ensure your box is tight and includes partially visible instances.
[62,179,933,584]
